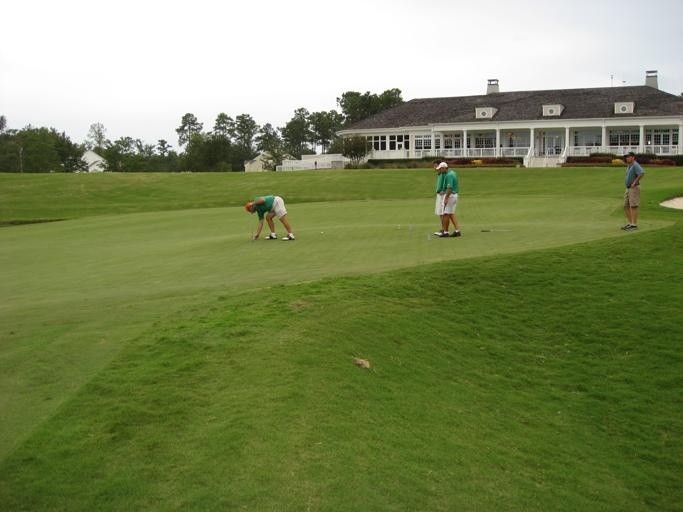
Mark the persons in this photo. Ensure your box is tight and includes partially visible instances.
[438,162,460,238]
[244,195,295,240]
[431,159,450,235]
[621,151,646,232]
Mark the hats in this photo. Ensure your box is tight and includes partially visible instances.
[436,161,448,170]
[624,152,634,156]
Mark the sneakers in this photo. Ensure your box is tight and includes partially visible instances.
[282,233,295,241]
[621,224,637,231]
[264,233,277,240]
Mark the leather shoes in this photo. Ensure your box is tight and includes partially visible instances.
[434,230,449,237]
[450,231,461,237]
